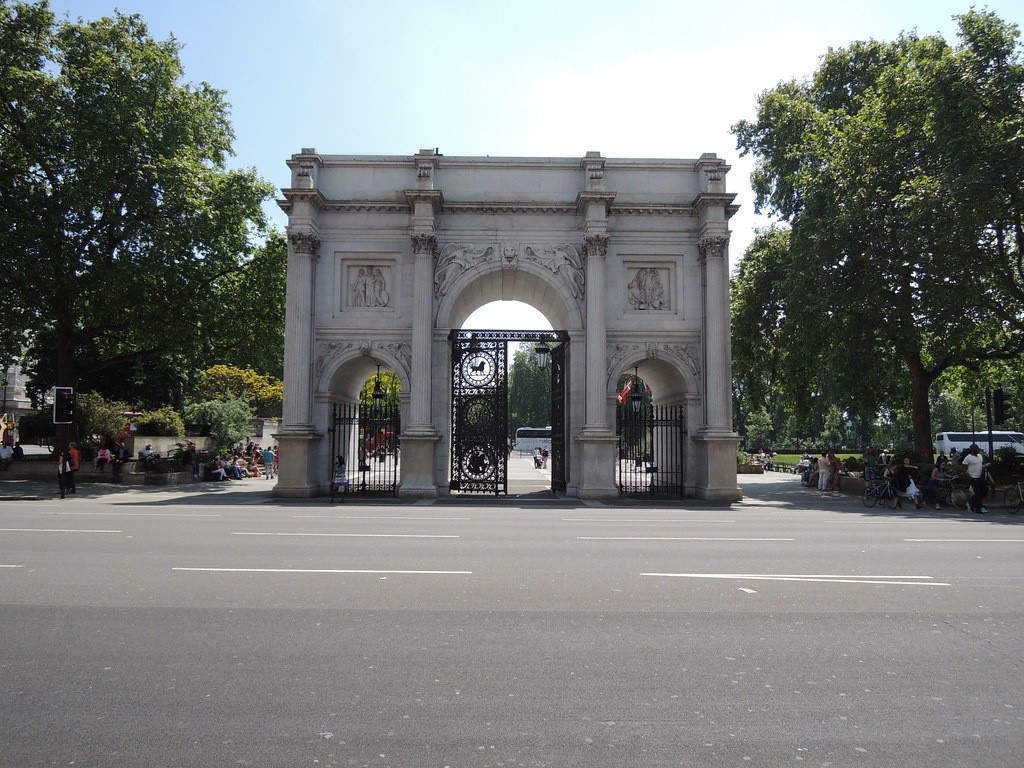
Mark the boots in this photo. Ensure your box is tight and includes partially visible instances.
[330,490,336,503]
[340,494,344,503]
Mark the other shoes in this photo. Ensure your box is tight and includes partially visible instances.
[970,504,975,512]
[981,508,990,512]
[975,509,984,514]
[897,501,903,509]
[916,504,923,509]
[936,507,943,510]
[801,482,807,486]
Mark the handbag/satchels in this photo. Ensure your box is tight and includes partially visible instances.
[338,484,344,492]
[59,461,71,473]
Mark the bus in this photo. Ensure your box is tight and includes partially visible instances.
[514,425,553,453]
[936,431,1024,454]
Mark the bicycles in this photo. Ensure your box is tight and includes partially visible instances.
[861,475,898,510]
[925,475,968,511]
[1003,480,1024,514]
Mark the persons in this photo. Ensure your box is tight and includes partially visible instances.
[541,446,549,469]
[898,457,920,510]
[95,444,110,472]
[961,444,989,515]
[863,447,877,490]
[818,453,831,491]
[113,443,129,474]
[66,442,79,494]
[138,443,153,457]
[533,447,540,469]
[6,442,23,471]
[0,441,14,472]
[330,455,349,504]
[927,460,958,510]
[755,447,999,501]
[210,441,279,481]
[182,446,202,479]
[56,440,71,499]
[360,444,401,465]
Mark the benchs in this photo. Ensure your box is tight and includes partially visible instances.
[205,466,219,481]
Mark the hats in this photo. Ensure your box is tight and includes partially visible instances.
[883,449,889,452]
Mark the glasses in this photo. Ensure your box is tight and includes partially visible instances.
[941,464,945,466]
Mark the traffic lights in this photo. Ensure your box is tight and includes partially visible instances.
[63,394,77,421]
[993,389,1013,425]
